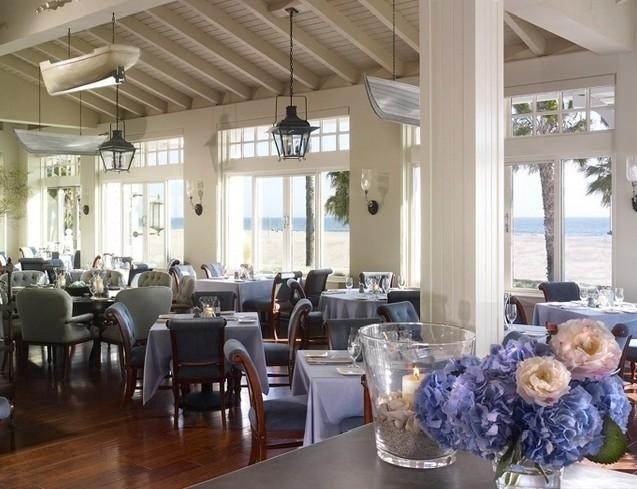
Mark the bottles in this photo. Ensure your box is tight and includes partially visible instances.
[213,296,220,317]
[234,272,239,279]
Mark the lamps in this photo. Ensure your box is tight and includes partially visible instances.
[75,193,89,215]
[185,179,203,217]
[267,6,321,162]
[94,83,142,174]
[625,155,636,209]
[360,167,379,215]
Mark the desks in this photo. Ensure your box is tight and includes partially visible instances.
[184,420,635,488]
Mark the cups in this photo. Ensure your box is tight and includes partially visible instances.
[358,322,477,471]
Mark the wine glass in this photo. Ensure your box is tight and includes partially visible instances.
[506,304,517,331]
[345,274,405,295]
[580,285,624,308]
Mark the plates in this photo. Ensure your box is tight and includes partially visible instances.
[605,309,619,314]
[527,331,546,337]
[234,279,244,282]
[335,367,365,376]
[238,319,256,323]
[306,351,328,358]
[220,311,233,314]
[622,309,637,313]
